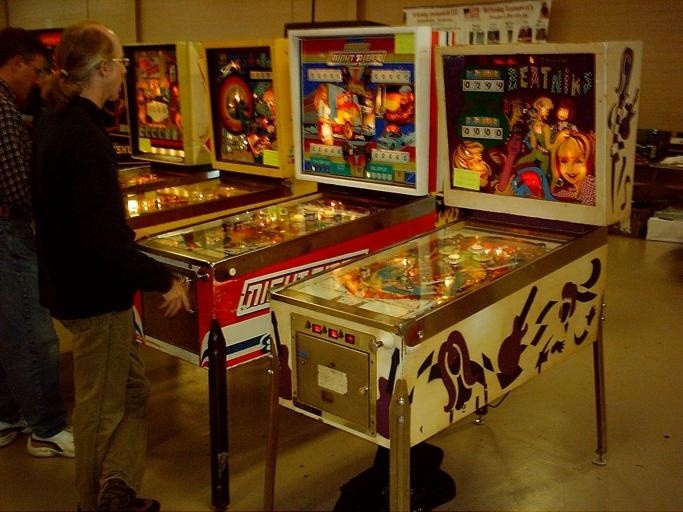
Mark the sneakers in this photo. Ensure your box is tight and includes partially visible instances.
[0,415,75,459]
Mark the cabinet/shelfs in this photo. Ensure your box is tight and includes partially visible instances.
[608,155,683,238]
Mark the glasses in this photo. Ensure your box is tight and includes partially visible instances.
[111,58,131,65]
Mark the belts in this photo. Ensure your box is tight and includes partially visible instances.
[0,205,28,218]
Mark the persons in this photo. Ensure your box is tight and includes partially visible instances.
[2,26,75,458]
[36,26,191,509]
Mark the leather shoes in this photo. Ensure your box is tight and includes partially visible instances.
[77,478,161,512]
